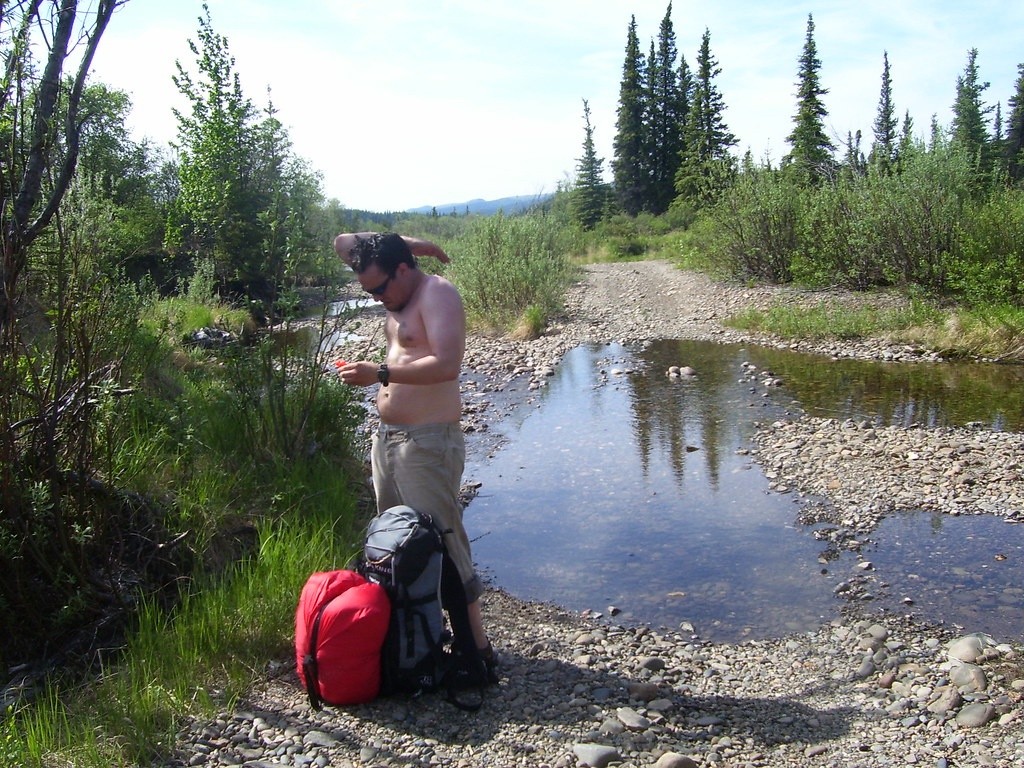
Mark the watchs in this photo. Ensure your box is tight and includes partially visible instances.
[377,364,389,388]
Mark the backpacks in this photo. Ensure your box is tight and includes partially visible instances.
[354,502,485,711]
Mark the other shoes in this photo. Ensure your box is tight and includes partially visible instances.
[441,640,497,692]
[438,617,452,644]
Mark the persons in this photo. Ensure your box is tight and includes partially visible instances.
[332,232,498,671]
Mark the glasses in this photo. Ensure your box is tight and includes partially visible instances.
[367,261,400,295]
